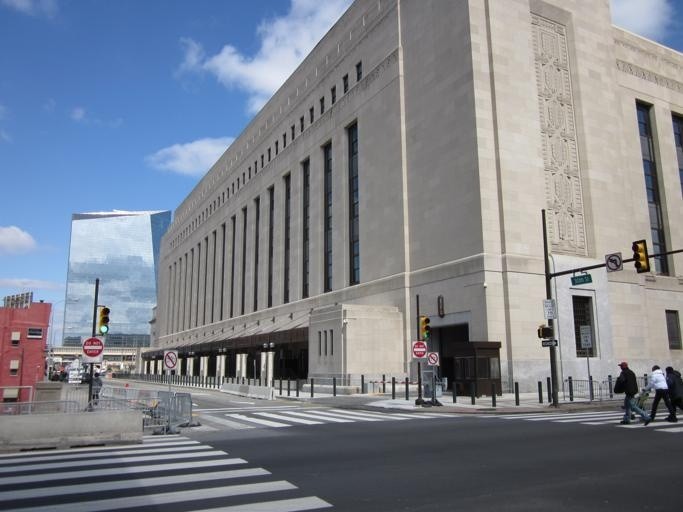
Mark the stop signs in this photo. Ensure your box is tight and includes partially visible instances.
[80,336,104,364]
[412,340,427,359]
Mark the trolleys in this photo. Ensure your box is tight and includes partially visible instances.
[621,388,651,420]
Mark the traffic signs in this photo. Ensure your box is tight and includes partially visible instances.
[570,274,591,285]
[541,340,557,347]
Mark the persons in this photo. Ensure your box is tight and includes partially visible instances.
[58,369,68,382]
[618,361,652,427]
[50,370,59,382]
[91,372,103,405]
[642,365,675,420]
[664,367,683,422]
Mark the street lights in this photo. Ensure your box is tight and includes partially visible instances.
[46,297,79,380]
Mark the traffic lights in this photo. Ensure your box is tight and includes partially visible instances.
[631,240,650,274]
[537,326,554,338]
[98,307,110,336]
[418,316,431,341]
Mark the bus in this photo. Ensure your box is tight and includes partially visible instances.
[51,360,101,379]
